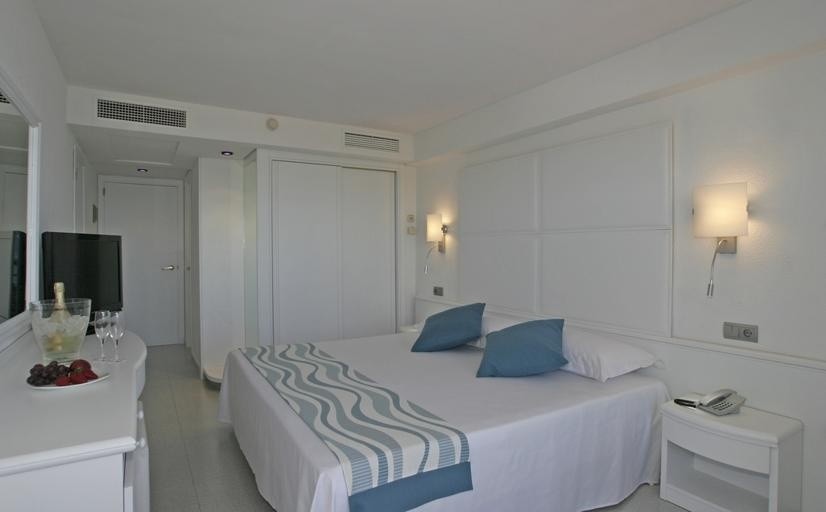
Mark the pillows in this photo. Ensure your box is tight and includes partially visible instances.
[411,302,656,382]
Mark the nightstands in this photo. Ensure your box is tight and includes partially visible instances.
[659,391,804,512]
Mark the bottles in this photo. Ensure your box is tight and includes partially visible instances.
[49,282,71,324]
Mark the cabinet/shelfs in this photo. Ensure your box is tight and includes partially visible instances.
[0,327,150,512]
[272,161,397,346]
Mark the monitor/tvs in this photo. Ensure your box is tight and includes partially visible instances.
[0,229,26,319]
[42,231,123,337]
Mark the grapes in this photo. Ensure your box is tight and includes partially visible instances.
[27,361,69,385]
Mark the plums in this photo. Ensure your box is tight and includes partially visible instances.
[70,359,91,370]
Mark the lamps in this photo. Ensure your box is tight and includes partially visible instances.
[692,182,749,296]
[424,214,447,274]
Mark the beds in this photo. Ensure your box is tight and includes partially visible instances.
[217,331,669,512]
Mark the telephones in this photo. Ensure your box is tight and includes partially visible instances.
[697,388,746,417]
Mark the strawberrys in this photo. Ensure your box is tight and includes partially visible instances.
[55,368,98,386]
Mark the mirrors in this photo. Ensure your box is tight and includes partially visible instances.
[0,91,29,325]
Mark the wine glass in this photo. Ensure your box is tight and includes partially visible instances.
[92,310,126,365]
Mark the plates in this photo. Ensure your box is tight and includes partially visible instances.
[25,368,112,390]
